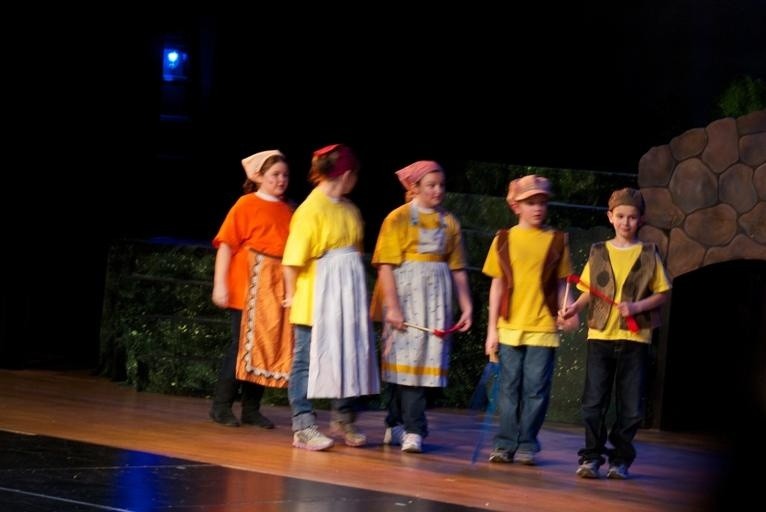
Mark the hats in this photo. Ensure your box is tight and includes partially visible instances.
[608,187,645,215]
[506,175,555,204]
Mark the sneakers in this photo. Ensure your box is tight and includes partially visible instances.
[489,448,512,462]
[606,463,629,478]
[512,449,534,464]
[383,425,405,445]
[329,421,368,446]
[294,428,334,450]
[576,459,600,478]
[401,432,423,452]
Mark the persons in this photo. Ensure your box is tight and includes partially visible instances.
[482,174,581,468]
[372,158,476,453]
[280,145,372,452]
[209,148,297,429]
[558,186,672,478]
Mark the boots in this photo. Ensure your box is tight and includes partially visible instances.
[208,379,240,427]
[241,380,273,429]
